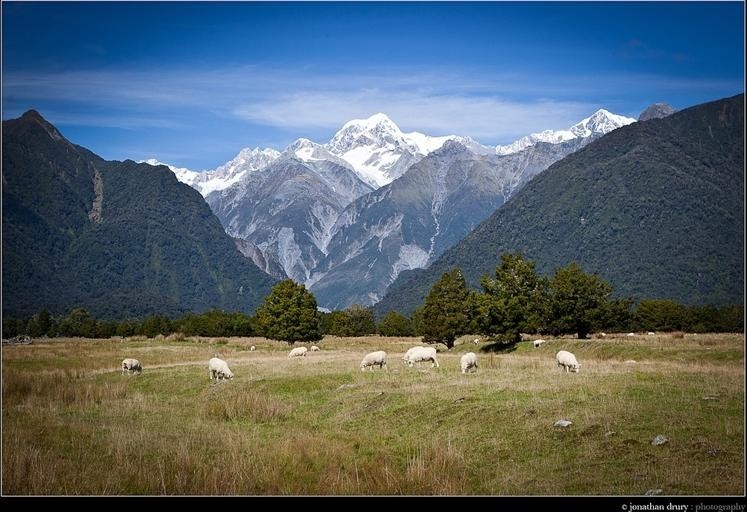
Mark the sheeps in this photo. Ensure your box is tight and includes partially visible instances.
[554,349,581,373]
[460,351,480,374]
[401,344,440,368]
[533,338,547,349]
[473,337,481,345]
[208,357,235,381]
[251,345,257,352]
[287,342,320,358]
[121,357,143,375]
[360,350,390,370]
